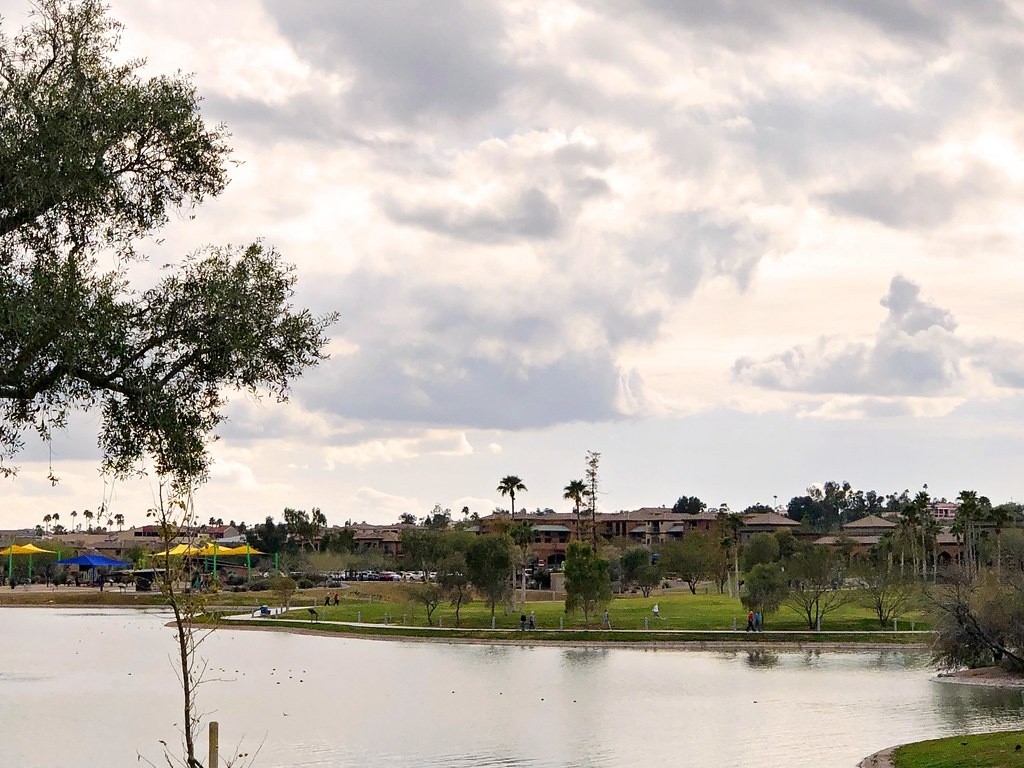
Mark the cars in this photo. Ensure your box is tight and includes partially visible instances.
[319,568,438,582]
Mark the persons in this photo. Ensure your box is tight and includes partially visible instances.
[333,590,340,606]
[831,578,840,591]
[323,592,331,606]
[788,579,792,591]
[800,579,805,591]
[746,609,756,632]
[652,603,660,618]
[738,579,742,591]
[528,612,536,630]
[599,609,613,630]
[755,612,763,632]
[519,613,527,632]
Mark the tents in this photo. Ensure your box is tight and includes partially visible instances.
[46,555,134,587]
[0,543,61,585]
[145,542,279,591]
